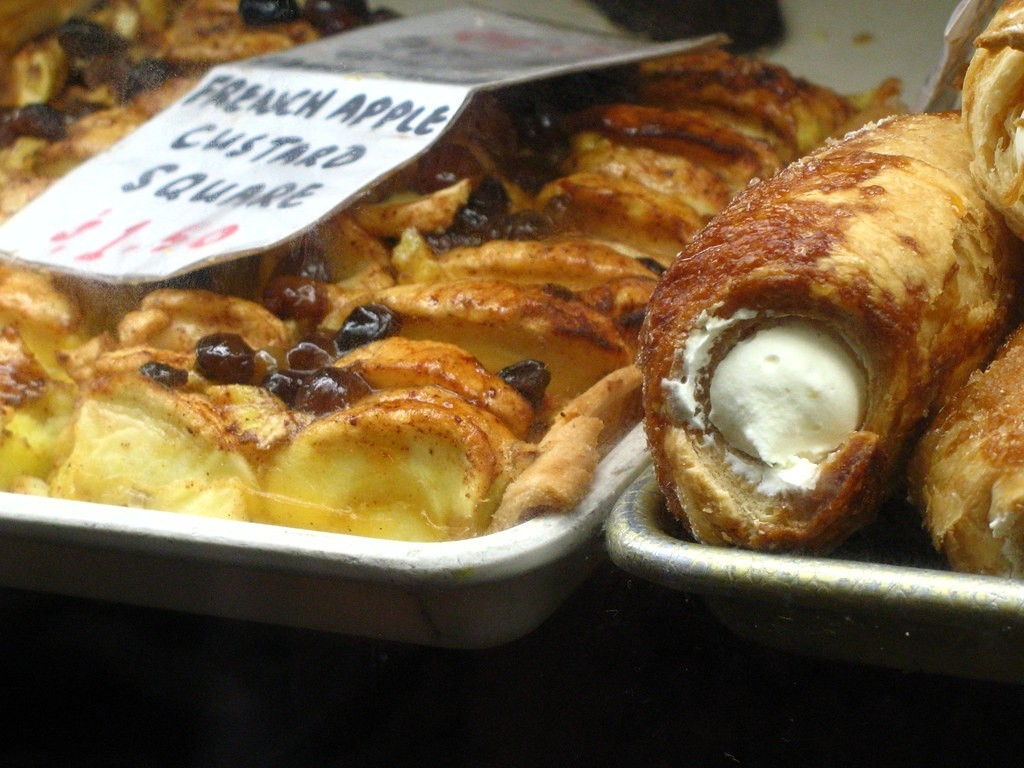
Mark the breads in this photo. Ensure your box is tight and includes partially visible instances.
[0,0,1024,578]
